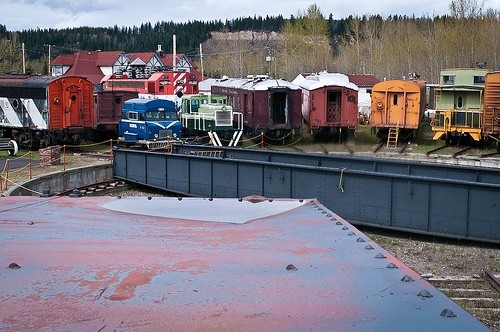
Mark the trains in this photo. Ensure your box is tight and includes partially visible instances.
[0,65,500,158]
